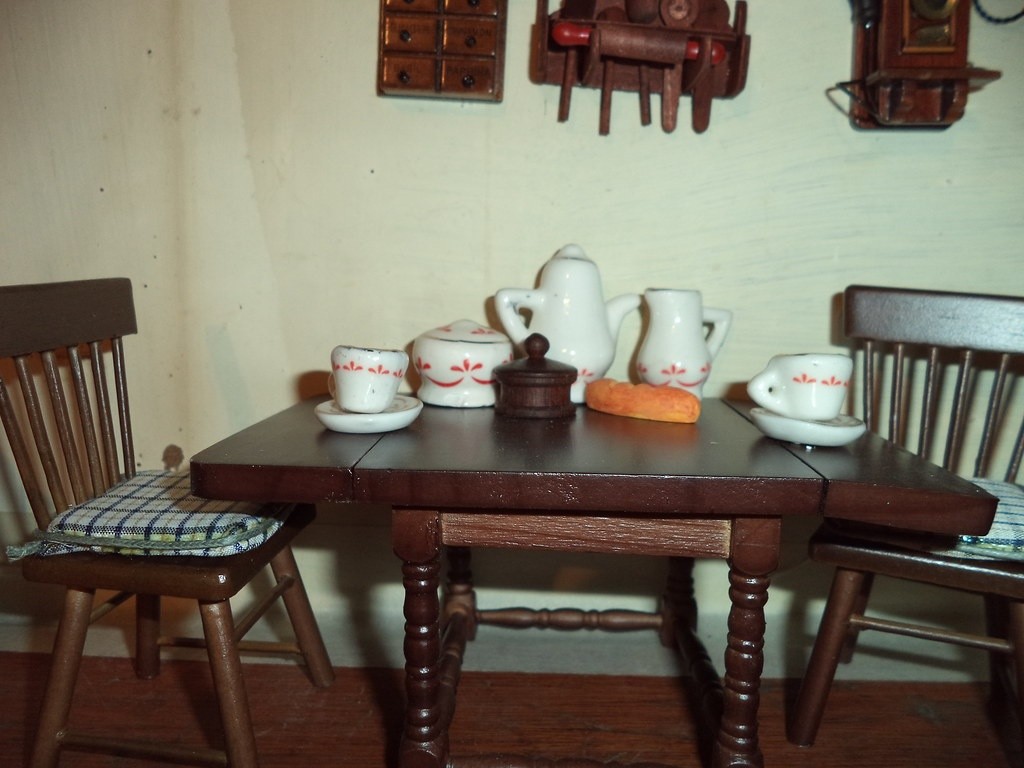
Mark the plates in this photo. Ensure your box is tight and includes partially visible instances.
[313,395,423,433]
[750,407,868,447]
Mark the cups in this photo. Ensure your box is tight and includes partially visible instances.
[747,354,855,420]
[327,346,409,413]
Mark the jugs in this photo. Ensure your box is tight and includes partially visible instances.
[636,288,733,402]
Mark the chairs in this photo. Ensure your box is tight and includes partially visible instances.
[798,286,1024,751]
[0,276,335,768]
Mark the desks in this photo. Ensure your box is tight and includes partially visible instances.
[190,391,1002,767]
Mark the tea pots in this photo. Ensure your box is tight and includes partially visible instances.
[494,244,642,403]
